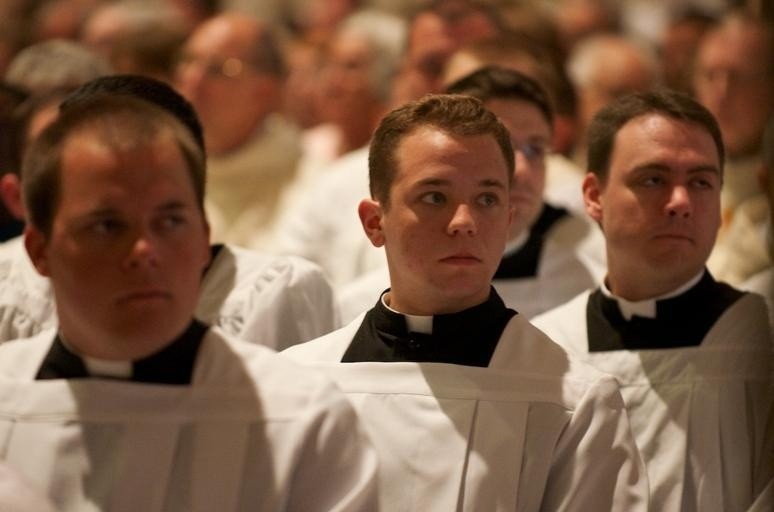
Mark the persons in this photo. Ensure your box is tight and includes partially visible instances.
[263,92,650,512]
[0,92,379,512]
[524,84,773,511]
[1,2,771,322]
